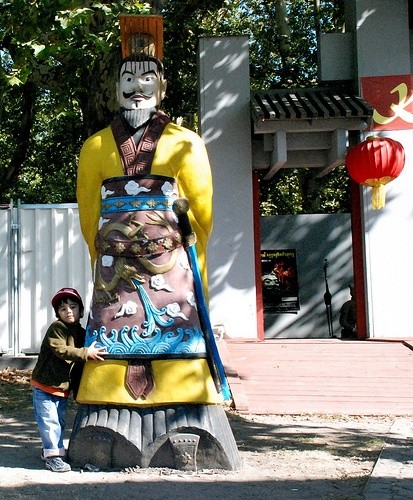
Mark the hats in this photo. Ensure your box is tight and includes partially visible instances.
[51,288,83,308]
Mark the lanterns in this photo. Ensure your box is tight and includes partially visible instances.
[345,135,405,210]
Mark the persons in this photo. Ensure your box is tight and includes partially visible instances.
[339,283,357,340]
[58,12,242,472]
[31,288,109,473]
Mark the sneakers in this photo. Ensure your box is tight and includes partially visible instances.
[41,447,45,459]
[45,457,71,472]
